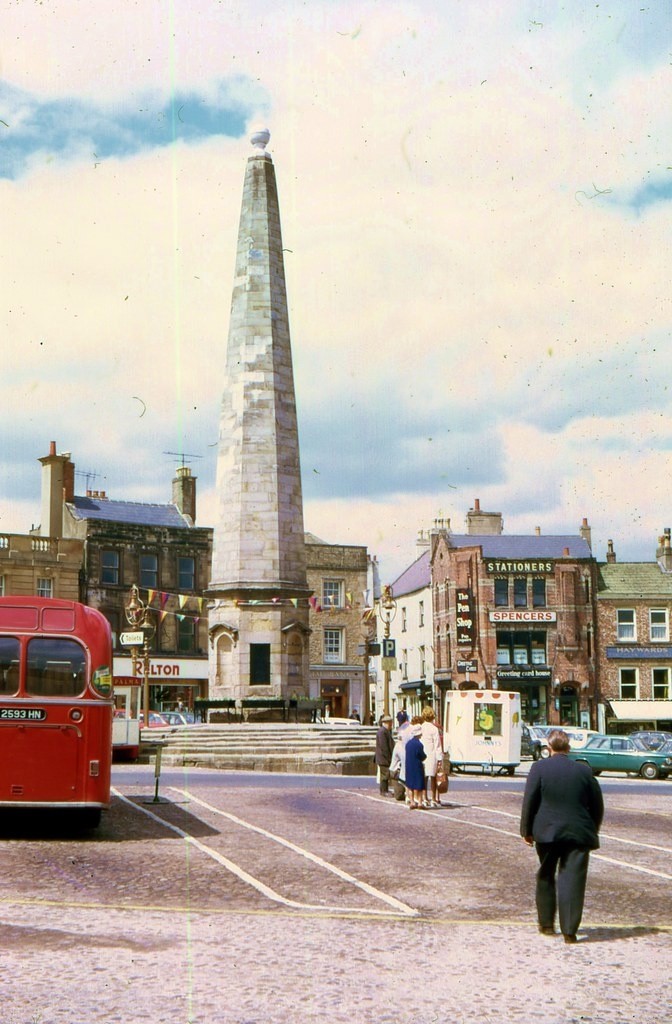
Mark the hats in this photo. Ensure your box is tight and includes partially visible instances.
[410,723,423,736]
[381,715,393,722]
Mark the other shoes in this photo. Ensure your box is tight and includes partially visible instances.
[410,804,417,809]
[539,926,556,936]
[380,791,394,797]
[418,804,425,809]
[564,933,577,944]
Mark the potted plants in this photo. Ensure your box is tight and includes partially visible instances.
[240,694,287,709]
[193,696,238,709]
[288,693,324,709]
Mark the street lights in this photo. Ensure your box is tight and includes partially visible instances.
[138,604,156,727]
[379,586,398,734]
[125,580,144,718]
[358,607,377,725]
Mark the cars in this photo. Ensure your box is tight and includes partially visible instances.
[114,702,202,729]
[522,724,672,761]
[569,734,672,780]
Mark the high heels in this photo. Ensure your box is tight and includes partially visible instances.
[423,800,430,806]
[430,801,441,808]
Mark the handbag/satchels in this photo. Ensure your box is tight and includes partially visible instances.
[435,765,448,793]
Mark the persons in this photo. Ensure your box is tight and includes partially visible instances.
[397,708,408,727]
[405,724,428,810]
[379,708,392,728]
[324,705,333,718]
[176,694,184,714]
[350,709,361,722]
[376,715,396,799]
[363,712,376,726]
[389,715,423,801]
[520,729,604,944]
[423,706,444,808]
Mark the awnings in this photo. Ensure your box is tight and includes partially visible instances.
[607,701,672,721]
[399,680,424,690]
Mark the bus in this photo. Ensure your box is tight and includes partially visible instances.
[0,595,115,828]
